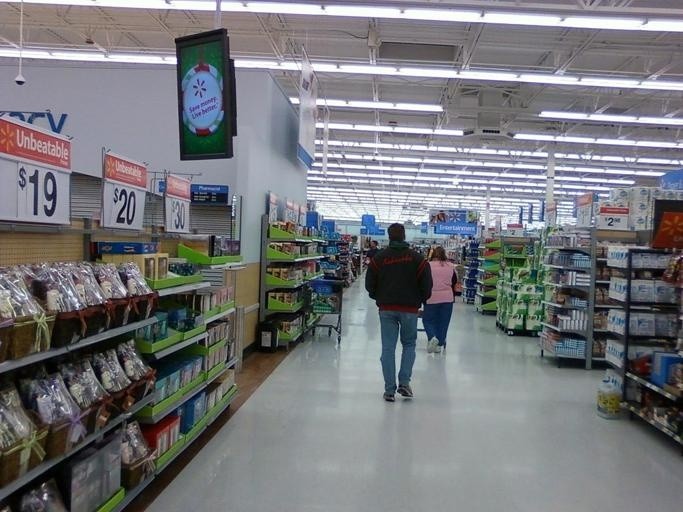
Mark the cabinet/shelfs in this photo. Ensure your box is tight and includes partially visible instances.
[537,234,682,446]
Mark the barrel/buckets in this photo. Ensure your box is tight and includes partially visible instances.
[596,379,621,419]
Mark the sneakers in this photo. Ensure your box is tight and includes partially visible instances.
[397,383,414,397]
[383,392,395,402]
[435,346,445,352]
[427,337,439,353]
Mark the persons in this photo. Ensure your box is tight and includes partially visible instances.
[364,222,434,401]
[365,240,378,266]
[422,245,457,355]
